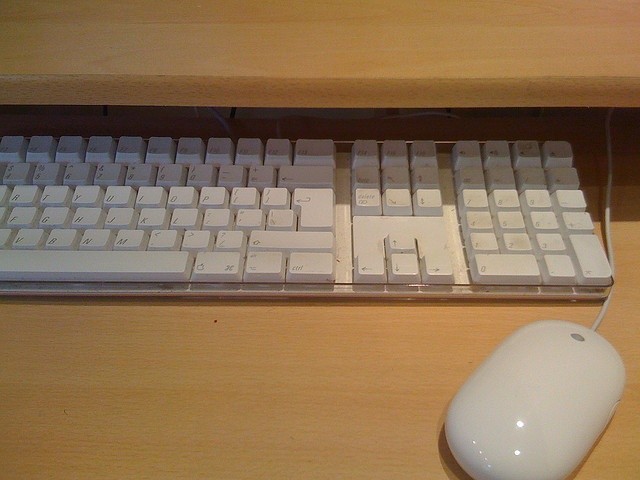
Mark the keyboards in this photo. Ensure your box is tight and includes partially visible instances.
[0,137,613,300]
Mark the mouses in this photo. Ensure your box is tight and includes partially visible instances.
[445,320,626,480]
